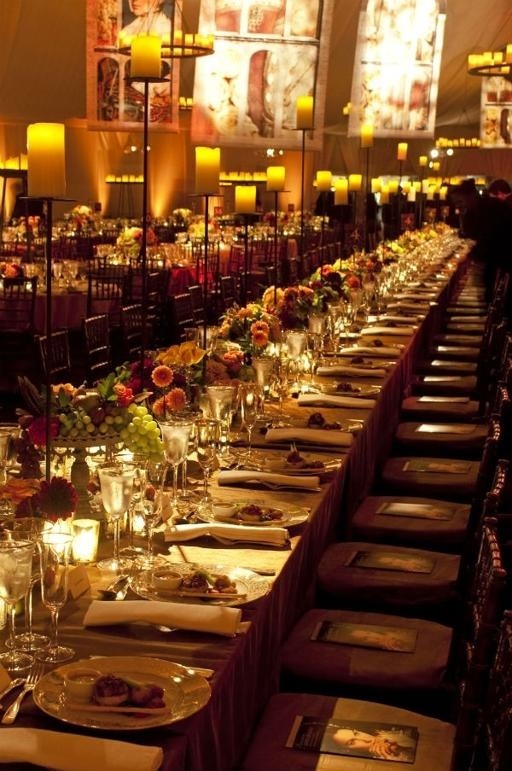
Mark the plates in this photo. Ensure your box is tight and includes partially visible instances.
[263,239,472,446]
[131,563,272,608]
[197,496,311,528]
[32,654,213,734]
[249,450,342,477]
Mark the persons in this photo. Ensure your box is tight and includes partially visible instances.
[338,623,414,649]
[124,1,171,37]
[186,214,223,245]
[329,721,419,763]
[0,210,156,254]
[422,463,471,474]
[449,177,512,276]
[484,179,512,214]
[368,552,433,573]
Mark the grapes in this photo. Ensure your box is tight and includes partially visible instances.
[58,404,165,456]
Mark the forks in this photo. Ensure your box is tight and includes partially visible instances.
[2,667,39,723]
[210,532,285,549]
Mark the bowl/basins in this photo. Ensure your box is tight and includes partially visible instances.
[264,455,288,469]
[60,667,103,704]
[209,501,237,518]
[152,569,184,592]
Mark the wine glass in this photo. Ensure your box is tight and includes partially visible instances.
[2,517,51,651]
[71,520,100,607]
[96,450,167,573]
[1,538,35,672]
[24,259,84,295]
[199,384,261,461]
[35,533,77,662]
[94,244,184,267]
[261,226,459,419]
[158,414,219,511]
